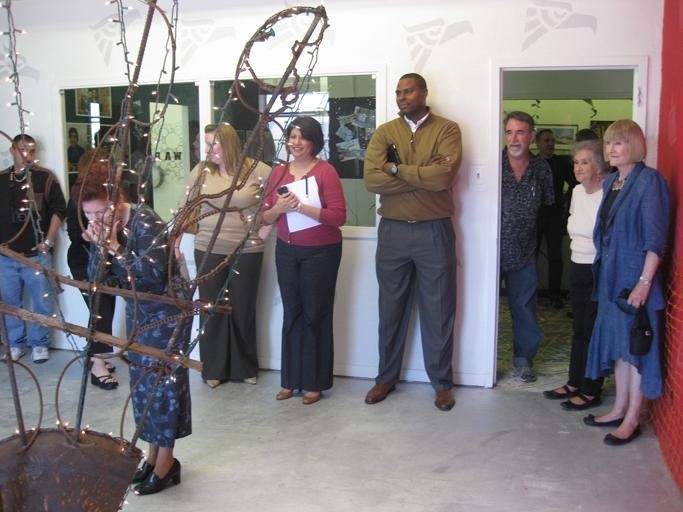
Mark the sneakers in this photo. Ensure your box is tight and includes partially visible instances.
[513,366,536,382]
[4,347,25,362]
[30,346,49,362]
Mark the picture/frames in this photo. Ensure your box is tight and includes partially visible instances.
[528,124,579,151]
[75,87,112,119]
[67,122,91,174]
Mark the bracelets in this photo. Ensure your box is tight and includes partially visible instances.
[42,238,54,250]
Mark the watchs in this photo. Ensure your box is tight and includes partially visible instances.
[388,162,401,177]
[638,276,650,287]
[295,201,302,213]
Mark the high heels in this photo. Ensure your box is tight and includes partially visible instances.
[134,456,180,494]
[276,388,302,400]
[86,359,115,372]
[129,460,158,484]
[90,372,118,390]
[303,390,323,404]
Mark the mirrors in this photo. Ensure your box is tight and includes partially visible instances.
[54,64,386,239]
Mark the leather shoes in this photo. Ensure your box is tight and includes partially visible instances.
[604,424,641,445]
[549,294,564,309]
[583,414,624,426]
[544,385,578,399]
[364,382,394,404]
[207,379,220,387]
[433,389,455,411]
[244,375,256,385]
[560,394,601,409]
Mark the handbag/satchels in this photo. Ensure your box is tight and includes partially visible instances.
[180,186,201,234]
[629,305,652,355]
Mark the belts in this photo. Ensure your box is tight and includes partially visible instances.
[0,252,38,258]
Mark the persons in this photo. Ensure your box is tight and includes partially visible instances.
[501,111,556,384]
[178,123,265,389]
[577,119,668,448]
[574,128,598,140]
[534,128,579,311]
[360,72,464,411]
[258,115,347,407]
[67,127,84,162]
[66,150,120,391]
[542,140,609,411]
[0,134,67,364]
[71,173,194,495]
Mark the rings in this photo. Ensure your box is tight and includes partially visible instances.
[291,204,294,208]
